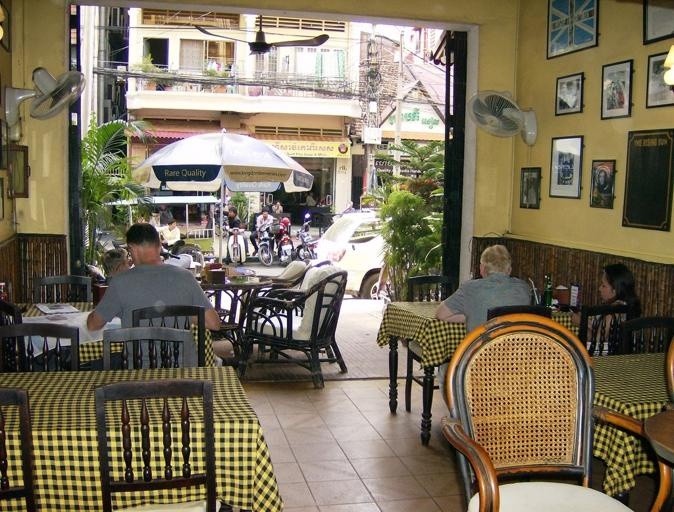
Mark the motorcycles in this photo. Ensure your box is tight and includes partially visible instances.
[223,223,247,266]
[256,221,274,266]
[295,211,318,260]
[275,216,296,264]
[114,238,204,274]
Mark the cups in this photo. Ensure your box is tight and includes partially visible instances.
[195,265,202,276]
[529,288,541,305]
[552,287,569,305]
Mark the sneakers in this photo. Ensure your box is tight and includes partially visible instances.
[252,248,259,256]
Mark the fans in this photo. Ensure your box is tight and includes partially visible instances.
[470,88,537,147]
[195,13,328,57]
[5,66,87,141]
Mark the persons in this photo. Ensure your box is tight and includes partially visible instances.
[86,222,225,331]
[215,207,249,263]
[435,243,533,411]
[598,170,607,189]
[250,205,275,255]
[155,217,181,248]
[306,193,318,207]
[272,201,283,213]
[568,262,641,342]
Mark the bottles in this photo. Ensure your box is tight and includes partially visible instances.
[543,276,552,306]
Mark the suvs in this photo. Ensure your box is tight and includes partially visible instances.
[318,212,395,300]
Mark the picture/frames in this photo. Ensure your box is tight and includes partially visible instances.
[646,50,674,110]
[642,0,674,46]
[546,0,598,60]
[0,118,9,170]
[600,57,632,120]
[520,166,541,209]
[0,0,11,53]
[588,158,616,208]
[554,71,584,116]
[0,176,5,222]
[549,135,583,199]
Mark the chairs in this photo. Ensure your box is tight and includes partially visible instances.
[1,255,351,303]
[405,274,452,413]
[246,270,349,389]
[1,322,81,372]
[578,303,638,355]
[1,304,25,369]
[439,313,671,512]
[102,327,196,367]
[0,387,39,512]
[618,314,671,352]
[131,305,206,367]
[94,378,234,512]
[486,305,553,320]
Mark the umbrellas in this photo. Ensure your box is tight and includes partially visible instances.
[131,129,315,263]
